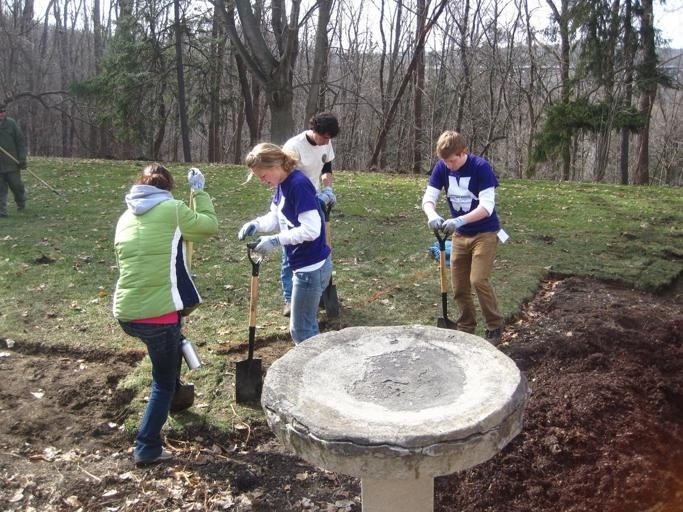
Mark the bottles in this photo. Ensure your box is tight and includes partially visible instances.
[178,334,202,371]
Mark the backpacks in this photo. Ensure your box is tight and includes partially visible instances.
[441,217,463,237]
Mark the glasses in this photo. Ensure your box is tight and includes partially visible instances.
[0,110,5,113]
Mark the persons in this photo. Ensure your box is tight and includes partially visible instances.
[238,142,333,348]
[0,103,27,218]
[279,112,340,317]
[111,162,219,466]
[421,130,504,346]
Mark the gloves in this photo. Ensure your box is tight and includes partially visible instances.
[187,167,205,192]
[17,159,27,170]
[237,222,256,241]
[316,191,329,205]
[321,187,336,208]
[253,233,279,256]
[425,213,445,230]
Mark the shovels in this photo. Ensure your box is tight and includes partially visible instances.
[171,169,195,412]
[320,195,341,318]
[236,242,262,401]
[434,224,458,329]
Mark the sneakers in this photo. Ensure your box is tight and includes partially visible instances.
[16,201,25,209]
[282,302,290,316]
[152,445,173,463]
[484,319,504,346]
[0,212,8,217]
